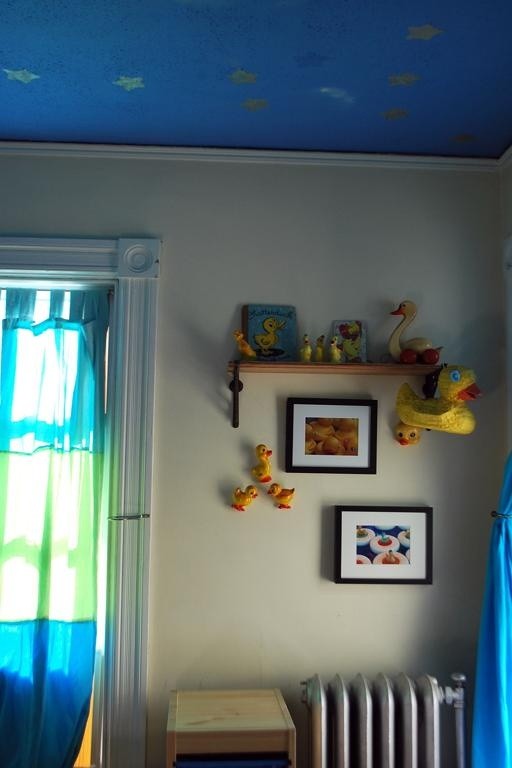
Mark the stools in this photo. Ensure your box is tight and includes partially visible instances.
[165,683,299,767]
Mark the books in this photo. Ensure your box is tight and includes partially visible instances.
[240,303,299,362]
[333,319,368,364]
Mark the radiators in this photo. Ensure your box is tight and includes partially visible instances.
[299,665,469,767]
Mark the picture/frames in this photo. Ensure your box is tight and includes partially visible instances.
[284,392,380,476]
[332,501,435,585]
